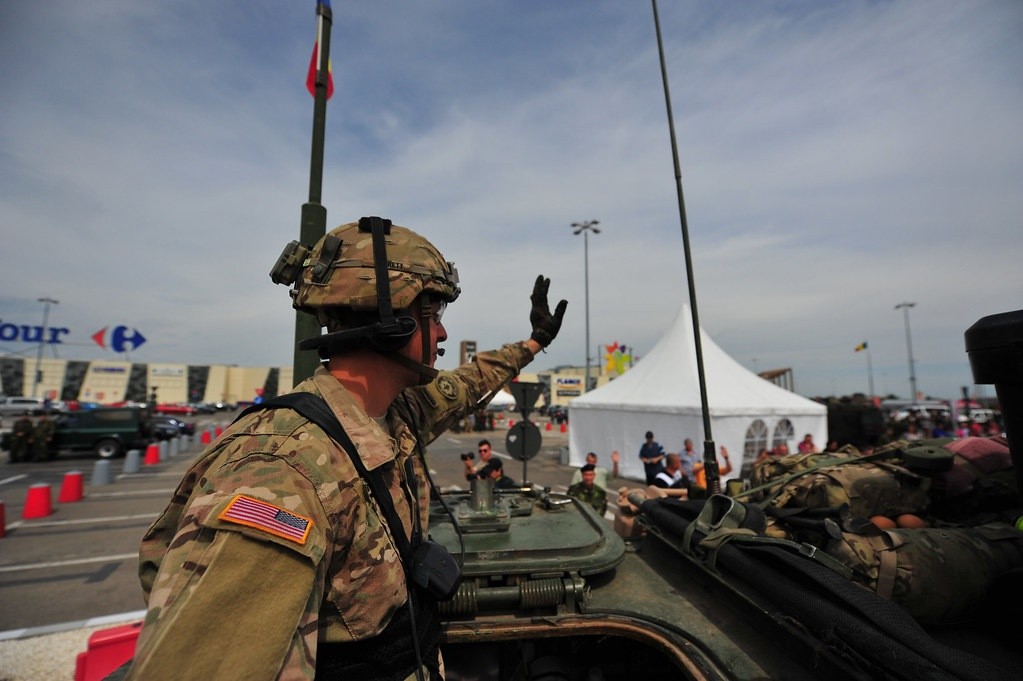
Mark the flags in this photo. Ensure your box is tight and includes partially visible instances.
[856,342,867,352]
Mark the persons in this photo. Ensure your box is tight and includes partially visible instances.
[823,440,838,453]
[639,431,666,486]
[568,464,608,519]
[896,421,1000,440]
[126,217,566,681]
[571,451,619,491]
[463,440,514,489]
[654,452,688,489]
[28,412,55,464]
[693,445,731,491]
[755,445,789,466]
[798,434,816,453]
[6,413,33,464]
[678,439,702,482]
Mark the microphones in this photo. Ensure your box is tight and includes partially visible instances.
[438,349,445,356]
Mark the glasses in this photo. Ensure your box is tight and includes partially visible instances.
[479,449,487,453]
[422,297,446,325]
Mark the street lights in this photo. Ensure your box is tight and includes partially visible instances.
[892,302,919,405]
[568,219,603,391]
[29,296,60,399]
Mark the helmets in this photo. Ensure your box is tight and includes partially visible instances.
[289,216,461,315]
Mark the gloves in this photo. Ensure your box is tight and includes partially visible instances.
[530,274,568,348]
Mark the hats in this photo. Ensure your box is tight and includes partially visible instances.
[485,458,502,473]
[646,432,654,438]
[581,464,594,472]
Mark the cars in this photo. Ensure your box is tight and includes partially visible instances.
[0,395,235,461]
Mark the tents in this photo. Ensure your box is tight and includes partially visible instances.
[568,305,829,488]
